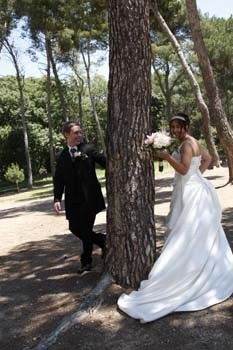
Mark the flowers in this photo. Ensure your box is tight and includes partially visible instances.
[144,130,173,150]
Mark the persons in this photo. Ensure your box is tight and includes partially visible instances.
[155,113,221,292]
[52,120,106,277]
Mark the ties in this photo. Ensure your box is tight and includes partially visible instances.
[69,149,75,162]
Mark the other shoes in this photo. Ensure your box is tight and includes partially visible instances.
[100,235,105,259]
[77,258,92,273]
